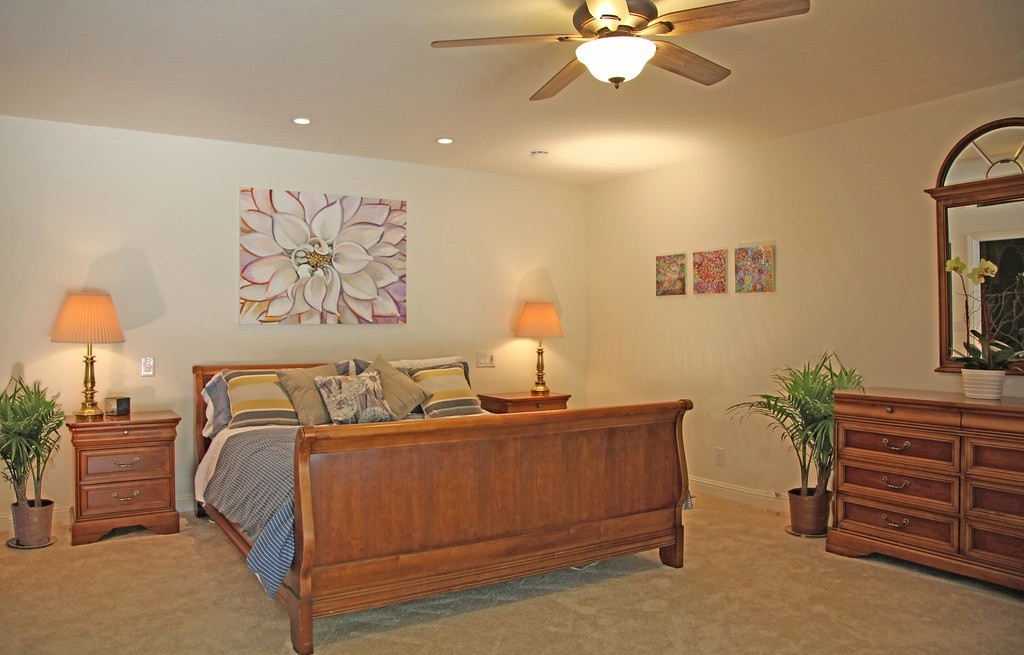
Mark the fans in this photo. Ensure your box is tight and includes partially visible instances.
[431,0,812,101]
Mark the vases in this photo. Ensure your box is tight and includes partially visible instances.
[961,367,1006,400]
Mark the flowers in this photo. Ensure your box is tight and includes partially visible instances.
[944,256,1015,369]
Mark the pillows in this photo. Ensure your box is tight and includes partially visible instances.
[201,359,480,439]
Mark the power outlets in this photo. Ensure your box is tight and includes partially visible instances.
[714,448,725,467]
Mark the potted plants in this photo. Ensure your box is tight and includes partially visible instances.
[727,349,864,536]
[0,370,67,548]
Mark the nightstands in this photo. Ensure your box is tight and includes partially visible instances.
[66,410,182,547]
[476,391,573,413]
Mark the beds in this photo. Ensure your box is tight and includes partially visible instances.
[190,360,694,654]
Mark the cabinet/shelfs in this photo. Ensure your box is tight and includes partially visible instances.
[824,385,1024,593]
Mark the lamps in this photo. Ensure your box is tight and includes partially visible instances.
[514,301,562,392]
[574,37,658,89]
[52,291,124,416]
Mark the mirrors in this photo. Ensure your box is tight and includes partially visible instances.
[924,173,1024,377]
